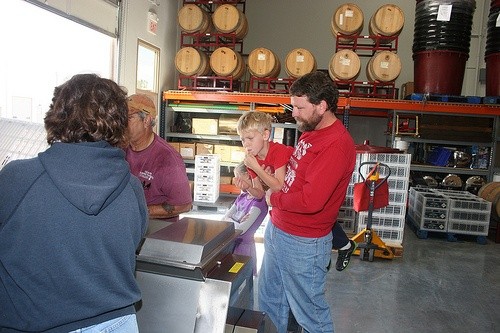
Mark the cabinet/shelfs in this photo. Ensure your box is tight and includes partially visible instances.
[160,91,298,207]
[386,110,500,192]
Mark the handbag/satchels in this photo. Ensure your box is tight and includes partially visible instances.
[353,162,389,212]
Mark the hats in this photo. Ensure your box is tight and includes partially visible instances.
[126,92,157,119]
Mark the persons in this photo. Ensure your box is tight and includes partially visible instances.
[221,161,268,277]
[258,71,357,333]
[233,111,356,272]
[0,74,150,333]
[125,94,192,219]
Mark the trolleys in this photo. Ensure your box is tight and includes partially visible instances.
[348,162,406,263]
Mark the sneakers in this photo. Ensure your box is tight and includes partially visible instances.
[336,240,356,271]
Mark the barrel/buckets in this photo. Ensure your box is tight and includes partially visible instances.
[247,48,281,80]
[477,182,500,220]
[210,47,247,80]
[411,0,500,96]
[369,4,405,44]
[177,4,213,39]
[329,3,364,43]
[212,4,249,40]
[285,48,318,80]
[328,49,361,85]
[174,47,213,77]
[366,50,402,82]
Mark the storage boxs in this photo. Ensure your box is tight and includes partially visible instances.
[407,187,492,235]
[167,141,246,163]
[337,152,411,244]
[428,148,452,167]
[187,175,241,198]
[191,112,241,137]
